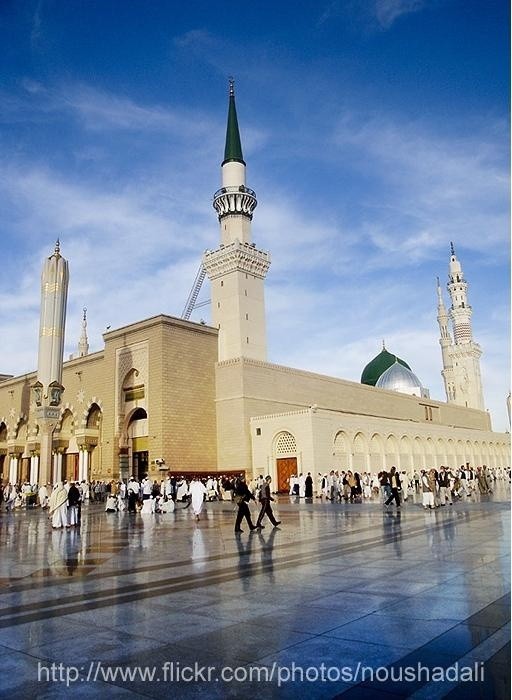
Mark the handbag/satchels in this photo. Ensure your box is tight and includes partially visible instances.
[234,496,243,503]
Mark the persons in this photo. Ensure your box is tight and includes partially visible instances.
[66,526,80,576]
[255,527,282,584]
[288,465,510,509]
[234,531,256,593]
[48,531,65,579]
[383,511,471,561]
[0,473,280,533]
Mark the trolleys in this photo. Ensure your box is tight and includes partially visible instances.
[22,491,39,509]
